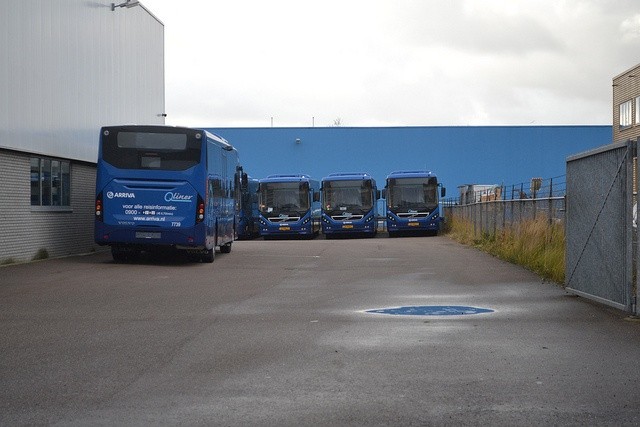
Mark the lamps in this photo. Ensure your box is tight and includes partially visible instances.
[111,0,141,11]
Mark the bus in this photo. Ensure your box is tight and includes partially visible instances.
[253,173,319,240]
[93,124,248,262]
[319,171,380,239]
[382,169,445,237]
[237,171,258,240]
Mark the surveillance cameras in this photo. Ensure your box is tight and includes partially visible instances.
[163,114,167,116]
[296,138,300,144]
